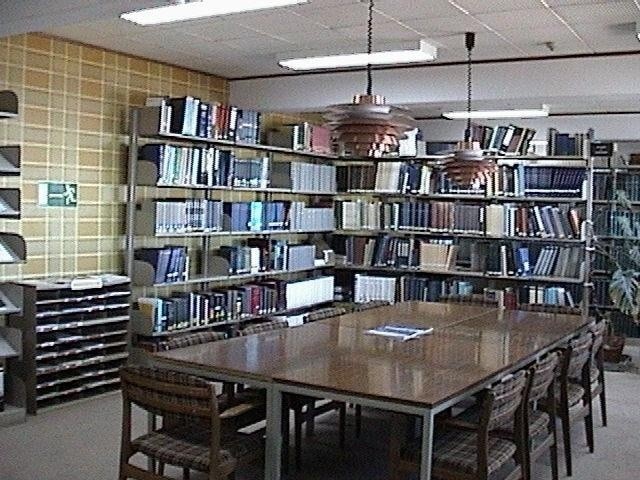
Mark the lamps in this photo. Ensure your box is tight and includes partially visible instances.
[119,0,314,27]
[276,41,437,71]
[438,33,503,190]
[441,104,549,120]
[324,2,415,161]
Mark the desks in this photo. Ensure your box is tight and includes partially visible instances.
[150,297,598,480]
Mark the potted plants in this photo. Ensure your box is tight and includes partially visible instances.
[594,192,640,363]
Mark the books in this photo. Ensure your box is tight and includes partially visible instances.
[340,125,640,339]
[125,94,340,334]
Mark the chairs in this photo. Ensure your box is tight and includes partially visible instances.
[390,371,530,480]
[582,319,608,446]
[149,332,289,480]
[536,333,595,476]
[355,300,391,309]
[235,319,344,467]
[120,367,265,479]
[446,350,563,480]
[303,308,361,438]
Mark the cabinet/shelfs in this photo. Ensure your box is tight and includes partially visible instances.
[0,89,27,429]
[337,139,592,315]
[594,165,640,337]
[6,275,129,418]
[120,111,336,344]
